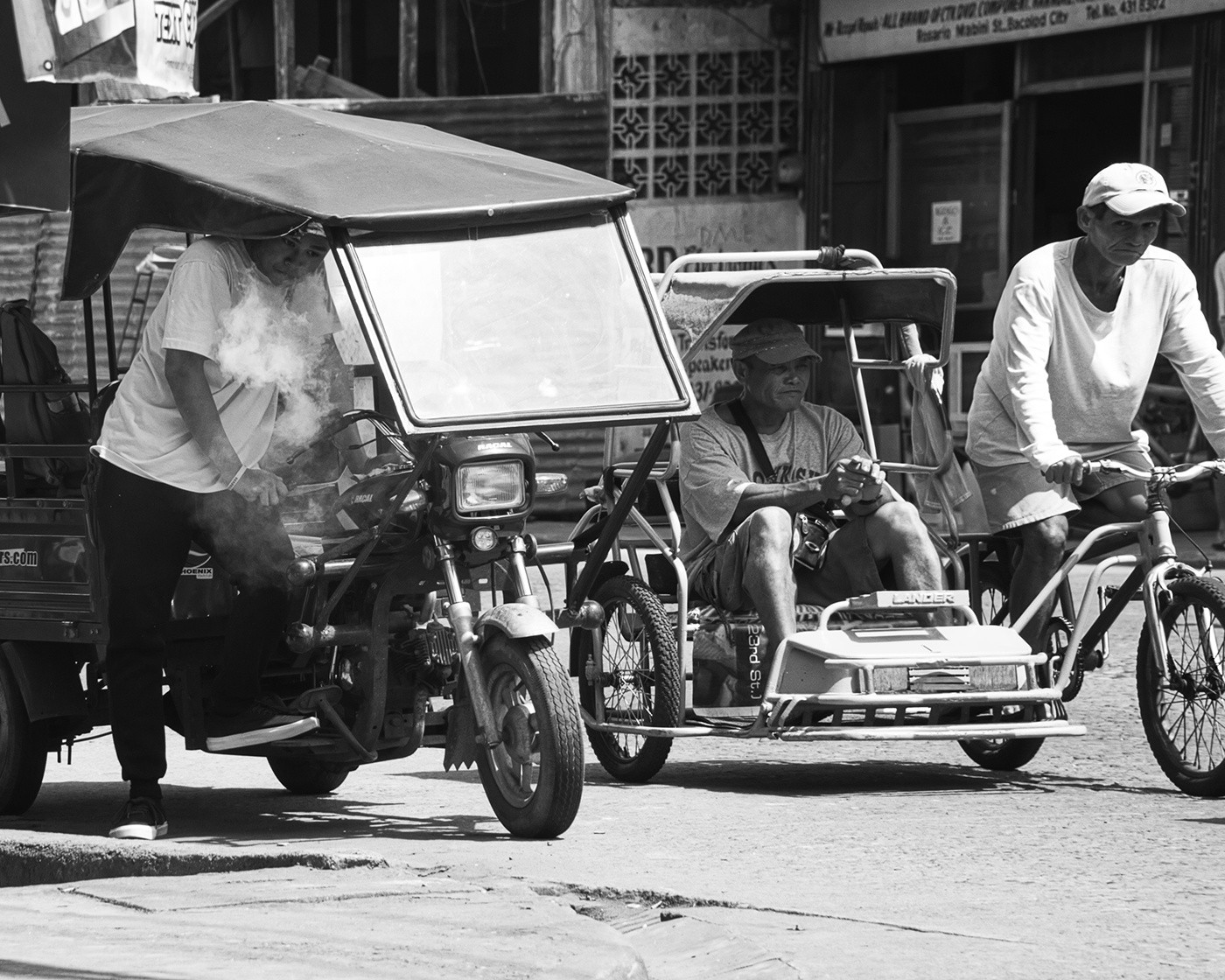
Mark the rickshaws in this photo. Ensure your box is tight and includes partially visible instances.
[553,245,1225,798]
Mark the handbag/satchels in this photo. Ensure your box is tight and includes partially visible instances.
[792,510,838,570]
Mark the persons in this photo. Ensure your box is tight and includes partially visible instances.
[964,162,1225,721]
[675,316,953,651]
[88,233,331,839]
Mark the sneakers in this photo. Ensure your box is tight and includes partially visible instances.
[207,702,321,751]
[109,796,168,839]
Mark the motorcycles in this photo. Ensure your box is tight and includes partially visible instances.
[1,97,703,840]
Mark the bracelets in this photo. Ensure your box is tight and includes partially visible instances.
[226,464,247,492]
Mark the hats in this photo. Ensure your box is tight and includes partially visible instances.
[729,318,823,363]
[1082,162,1187,217]
